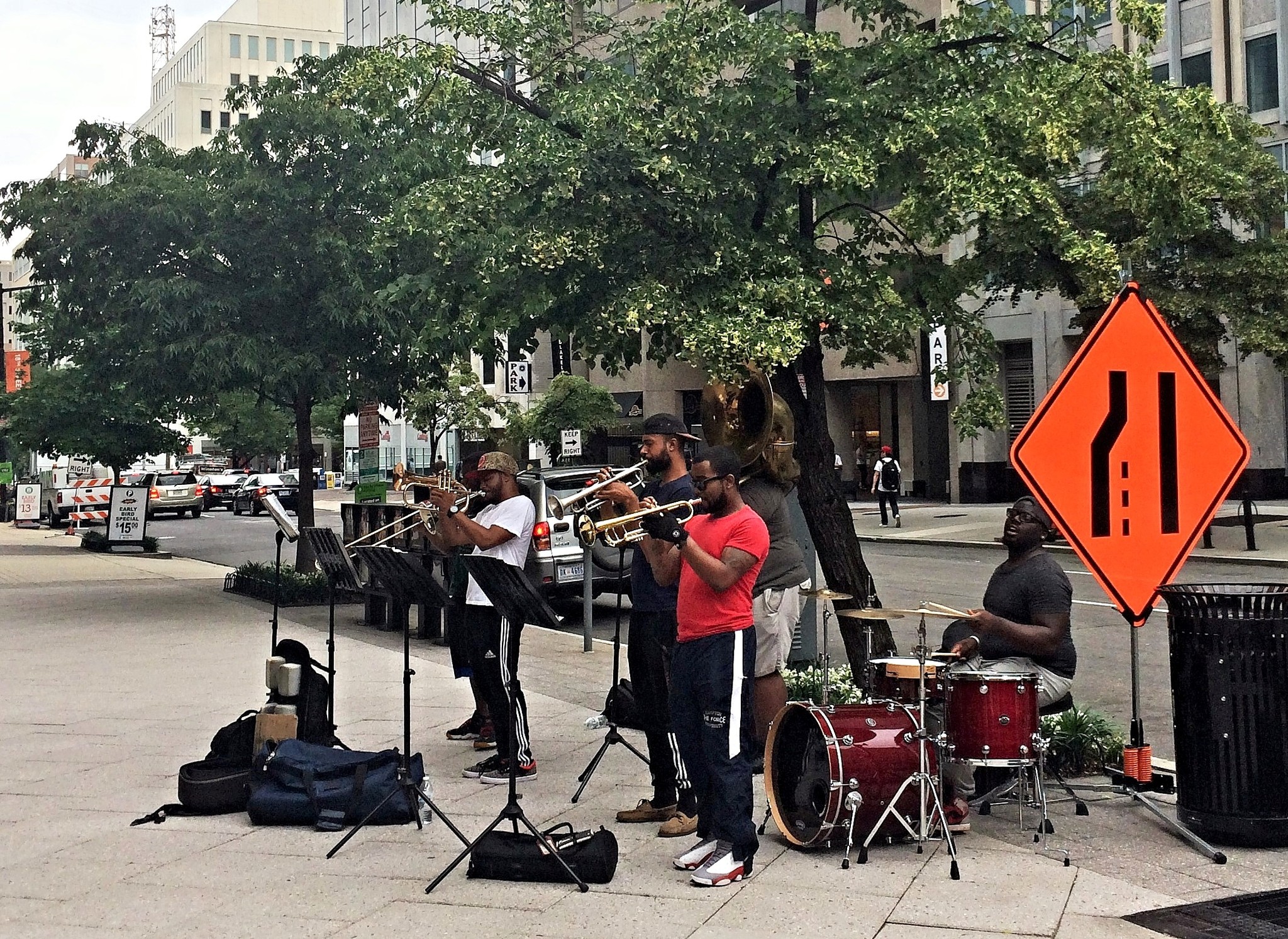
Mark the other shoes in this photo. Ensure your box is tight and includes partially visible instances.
[931,797,969,824]
[895,515,901,528]
[879,523,888,527]
[859,485,868,490]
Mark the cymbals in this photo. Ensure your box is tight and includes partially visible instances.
[834,607,905,620]
[798,588,854,600]
[869,608,980,621]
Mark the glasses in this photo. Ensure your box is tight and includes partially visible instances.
[1006,508,1050,533]
[692,472,738,491]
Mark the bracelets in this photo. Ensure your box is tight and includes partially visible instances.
[970,636,980,647]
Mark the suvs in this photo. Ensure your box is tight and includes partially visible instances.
[122,459,260,520]
[521,464,643,604]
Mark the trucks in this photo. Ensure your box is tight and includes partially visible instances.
[41,465,129,528]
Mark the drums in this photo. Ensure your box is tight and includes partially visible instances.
[763,699,939,849]
[944,670,1042,767]
[865,657,948,699]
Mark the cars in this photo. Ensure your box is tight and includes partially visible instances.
[232,473,298,517]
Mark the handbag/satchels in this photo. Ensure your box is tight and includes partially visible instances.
[467,821,618,884]
[243,736,426,831]
[600,678,646,731]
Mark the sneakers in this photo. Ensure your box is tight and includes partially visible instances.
[616,798,678,822]
[672,831,718,870]
[446,710,487,739]
[690,838,754,886]
[462,754,510,777]
[658,810,699,837]
[474,717,497,748]
[481,758,538,784]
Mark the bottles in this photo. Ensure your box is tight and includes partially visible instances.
[418,777,434,824]
[584,715,608,729]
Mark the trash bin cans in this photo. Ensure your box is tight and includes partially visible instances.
[318,472,343,490]
[1156,582,1288,848]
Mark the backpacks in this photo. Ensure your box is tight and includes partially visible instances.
[204,709,264,761]
[879,458,900,490]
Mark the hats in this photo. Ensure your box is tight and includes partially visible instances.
[1014,496,1056,542]
[641,412,702,443]
[882,445,892,455]
[461,451,484,477]
[477,451,520,477]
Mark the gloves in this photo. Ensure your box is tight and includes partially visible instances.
[640,512,688,549]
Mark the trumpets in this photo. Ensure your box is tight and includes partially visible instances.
[392,462,486,514]
[577,496,702,547]
[547,458,649,521]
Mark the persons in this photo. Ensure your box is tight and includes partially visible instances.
[856,443,868,490]
[639,446,771,887]
[834,454,843,478]
[591,413,704,837]
[930,495,1077,826]
[408,451,535,783]
[737,475,814,772]
[871,445,902,528]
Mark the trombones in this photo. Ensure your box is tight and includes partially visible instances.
[313,469,527,571]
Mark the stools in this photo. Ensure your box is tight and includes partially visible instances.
[1036,691,1082,779]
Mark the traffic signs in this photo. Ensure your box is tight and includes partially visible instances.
[561,429,582,457]
[68,453,91,475]
[928,310,949,401]
[506,361,527,393]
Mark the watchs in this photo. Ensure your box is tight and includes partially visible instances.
[447,505,460,518]
